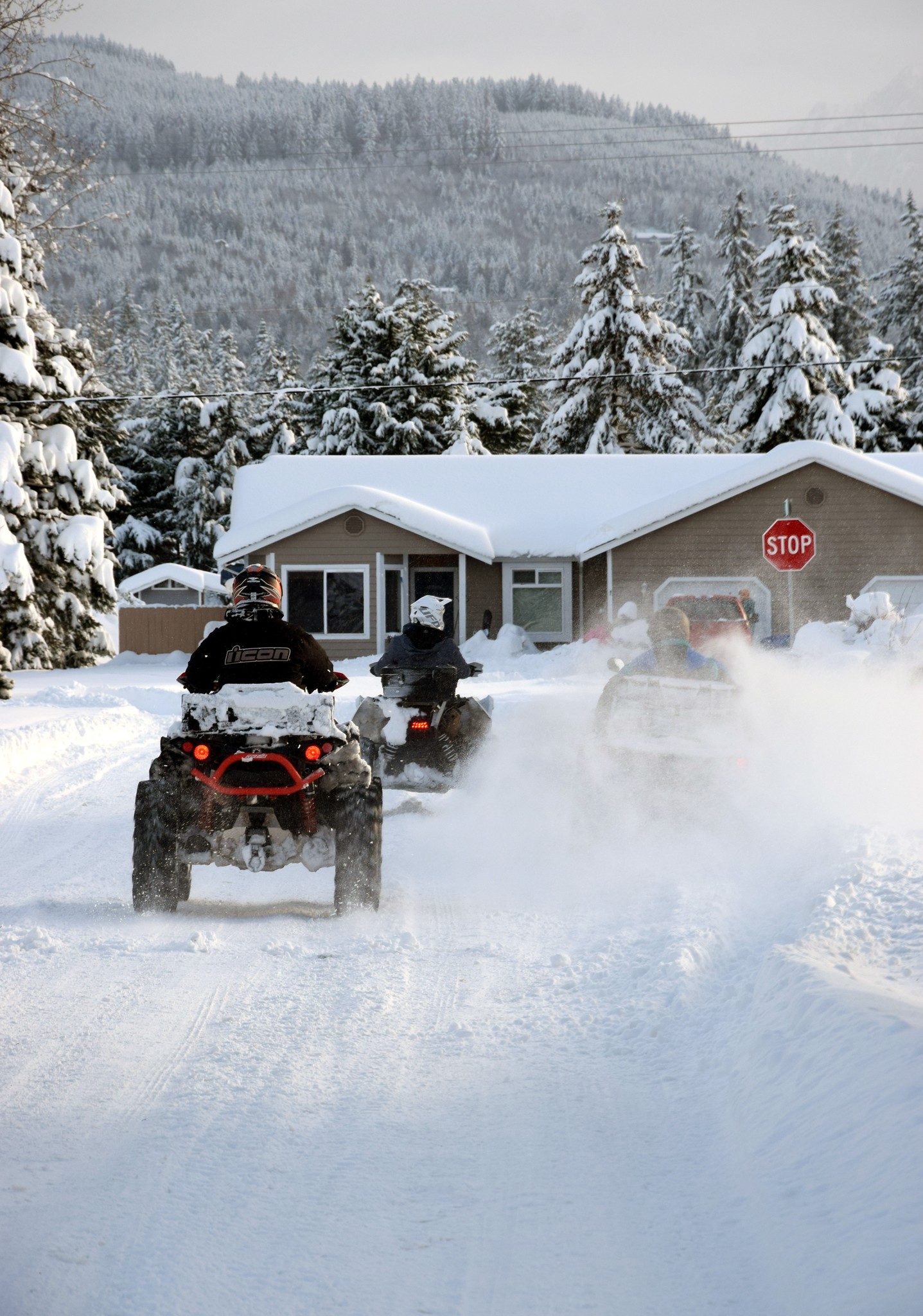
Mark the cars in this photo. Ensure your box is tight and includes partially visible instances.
[666,593,759,652]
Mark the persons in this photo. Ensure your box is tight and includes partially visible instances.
[617,607,731,684]
[739,589,755,635]
[370,595,484,680]
[185,565,339,694]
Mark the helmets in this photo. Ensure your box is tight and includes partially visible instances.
[410,595,452,630]
[232,563,283,609]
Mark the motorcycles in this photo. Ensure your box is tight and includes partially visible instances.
[593,658,740,763]
[351,661,492,793]
[131,672,384,918]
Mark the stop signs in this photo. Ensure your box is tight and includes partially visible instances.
[761,517,817,572]
